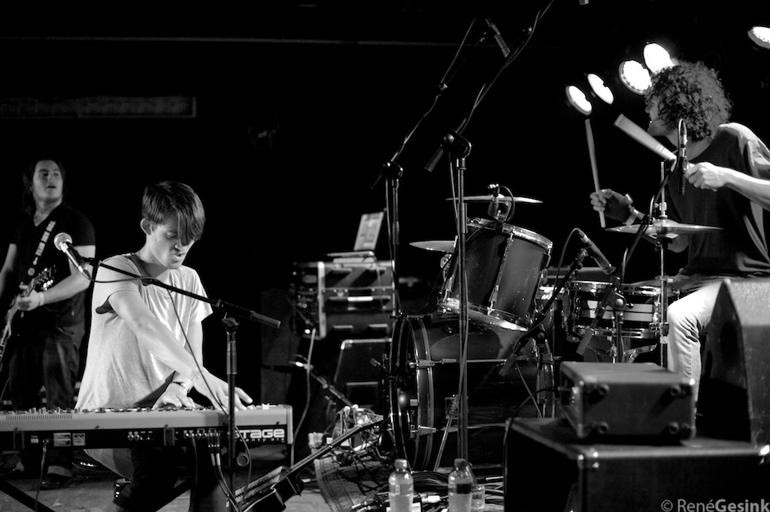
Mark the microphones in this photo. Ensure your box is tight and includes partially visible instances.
[487,22,511,57]
[678,120,688,195]
[580,234,616,275]
[579,0,589,5]
[54,232,90,279]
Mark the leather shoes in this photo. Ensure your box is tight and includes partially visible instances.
[40,474,71,489]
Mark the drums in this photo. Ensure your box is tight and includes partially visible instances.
[569,281,666,340]
[436,216,554,333]
[390,317,554,475]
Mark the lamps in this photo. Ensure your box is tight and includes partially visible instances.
[560,13,770,117]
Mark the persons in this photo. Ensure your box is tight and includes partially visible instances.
[590,61,770,440]
[0,154,97,485]
[72,179,254,512]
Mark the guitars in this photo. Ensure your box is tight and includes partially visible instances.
[1,269,53,358]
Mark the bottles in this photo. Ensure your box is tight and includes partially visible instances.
[471,483,485,512]
[387,458,413,512]
[448,457,474,512]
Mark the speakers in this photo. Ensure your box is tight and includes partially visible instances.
[502,416,770,512]
[697,280,770,444]
[557,361,693,443]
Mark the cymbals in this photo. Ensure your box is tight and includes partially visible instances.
[447,196,542,203]
[408,241,456,252]
[607,223,721,235]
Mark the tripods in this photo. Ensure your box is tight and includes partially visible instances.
[414,158,504,512]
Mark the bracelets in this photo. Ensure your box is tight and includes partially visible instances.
[39,292,45,306]
